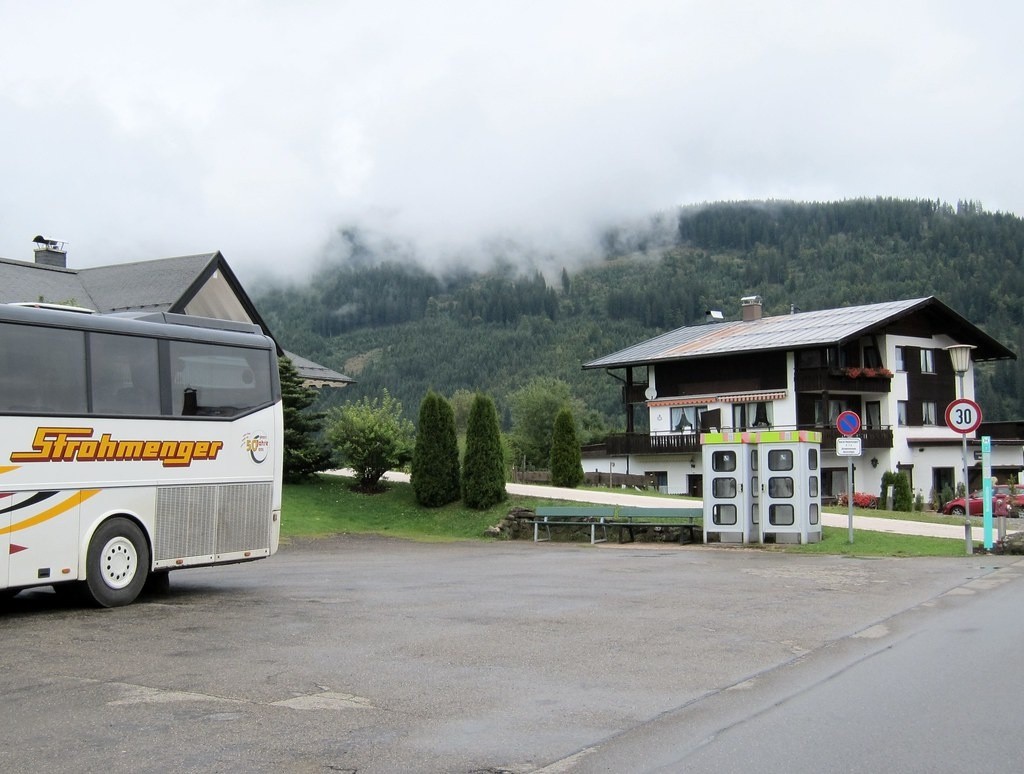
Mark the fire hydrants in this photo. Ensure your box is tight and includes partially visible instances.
[994,493,1012,542]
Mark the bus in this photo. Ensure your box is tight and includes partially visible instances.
[0,299,284,613]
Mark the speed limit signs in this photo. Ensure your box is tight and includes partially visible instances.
[944,399,983,433]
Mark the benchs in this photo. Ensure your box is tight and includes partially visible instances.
[609,507,703,546]
[526,506,615,544]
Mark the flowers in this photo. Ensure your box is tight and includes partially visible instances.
[847,367,862,379]
[875,367,892,378]
[862,368,876,378]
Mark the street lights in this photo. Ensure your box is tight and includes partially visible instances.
[942,345,979,555]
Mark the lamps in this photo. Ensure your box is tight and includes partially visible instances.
[870,455,879,468]
[689,458,695,468]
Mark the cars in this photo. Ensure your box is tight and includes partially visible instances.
[943,483,1024,517]
